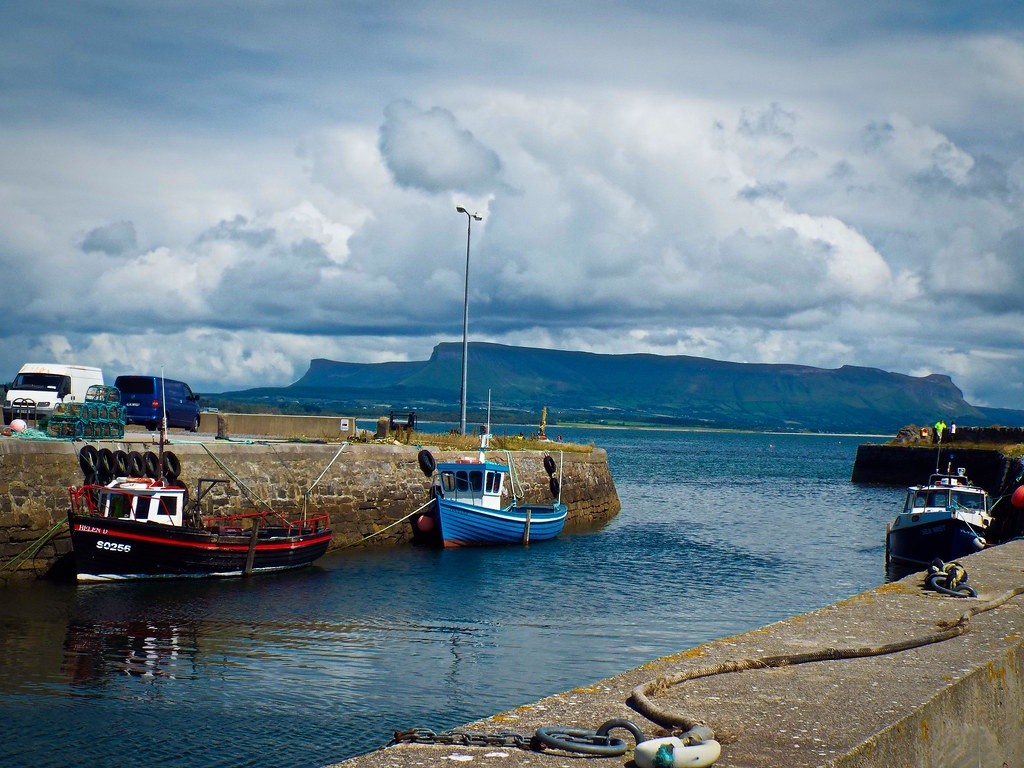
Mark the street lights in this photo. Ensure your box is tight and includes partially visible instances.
[455,202,484,440]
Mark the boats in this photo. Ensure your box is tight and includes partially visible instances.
[406,435,569,547]
[885,452,998,568]
[66,366,334,574]
[387,409,418,435]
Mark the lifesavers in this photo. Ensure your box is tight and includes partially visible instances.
[542,453,559,477]
[933,478,964,488]
[75,444,183,480]
[454,457,482,465]
[416,448,437,472]
[548,477,562,497]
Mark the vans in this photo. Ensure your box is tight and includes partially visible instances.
[3,363,104,424]
[112,374,202,432]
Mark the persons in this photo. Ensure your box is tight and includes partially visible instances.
[949,421,956,444]
[934,418,947,445]
